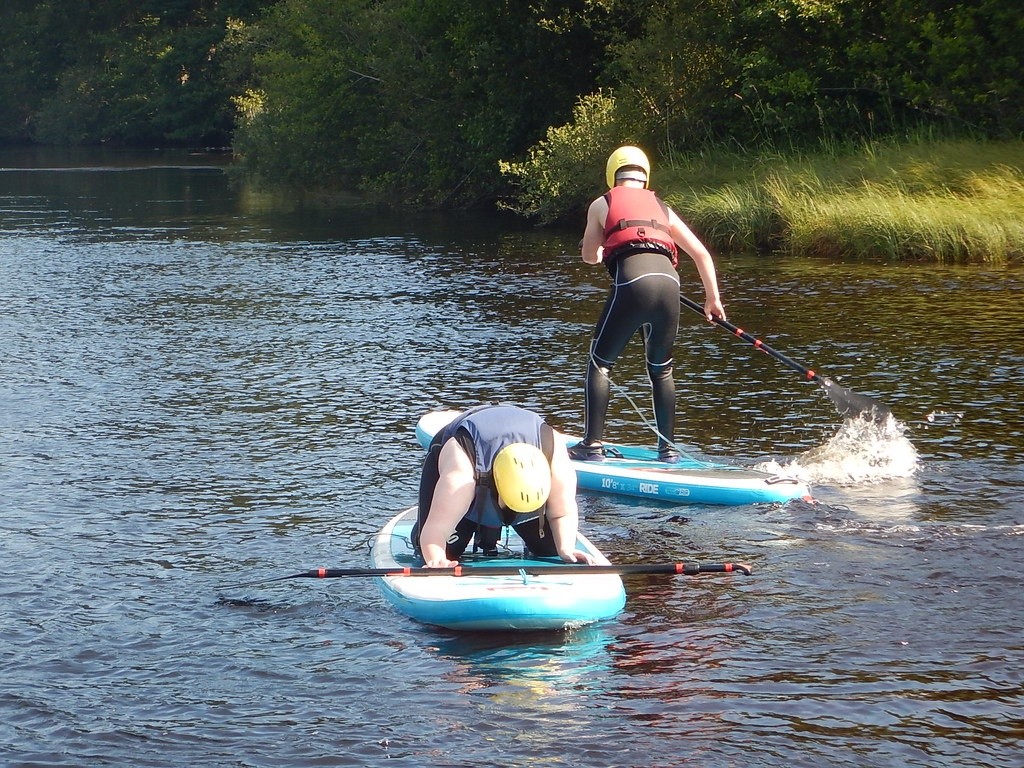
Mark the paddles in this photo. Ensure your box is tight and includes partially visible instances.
[680,296,891,430]
[210,564,756,592]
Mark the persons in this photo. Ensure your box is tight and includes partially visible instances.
[410,403,597,571]
[565,147,730,463]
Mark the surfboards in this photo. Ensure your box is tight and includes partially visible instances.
[369,492,629,633]
[414,402,815,505]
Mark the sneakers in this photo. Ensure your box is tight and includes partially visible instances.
[567,441,604,461]
[658,448,679,463]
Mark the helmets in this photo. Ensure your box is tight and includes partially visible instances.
[606,146,650,189]
[493,443,550,511]
